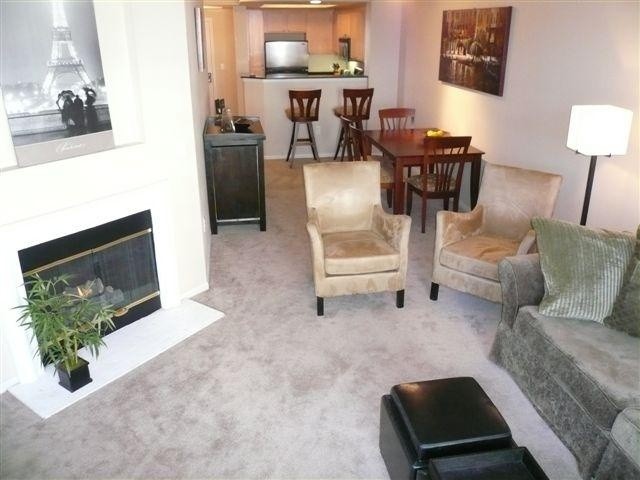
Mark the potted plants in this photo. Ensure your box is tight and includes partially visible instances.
[12,272,118,392]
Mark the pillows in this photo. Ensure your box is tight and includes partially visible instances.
[533,217,640,339]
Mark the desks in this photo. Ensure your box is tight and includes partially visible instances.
[362,129,485,229]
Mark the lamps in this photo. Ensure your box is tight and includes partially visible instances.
[567,105,634,226]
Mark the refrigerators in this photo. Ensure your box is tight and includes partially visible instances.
[264,41,309,79]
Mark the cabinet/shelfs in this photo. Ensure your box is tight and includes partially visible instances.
[204,115,266,235]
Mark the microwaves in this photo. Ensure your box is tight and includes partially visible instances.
[338,38,350,62]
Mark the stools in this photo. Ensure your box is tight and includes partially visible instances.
[380,377,518,480]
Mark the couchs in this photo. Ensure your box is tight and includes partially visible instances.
[489,223,640,480]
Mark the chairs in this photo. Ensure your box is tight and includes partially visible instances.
[334,89,373,161]
[349,126,406,216]
[430,163,563,303]
[302,160,412,316]
[340,115,366,161]
[285,90,322,168]
[407,137,471,233]
[378,108,414,178]
